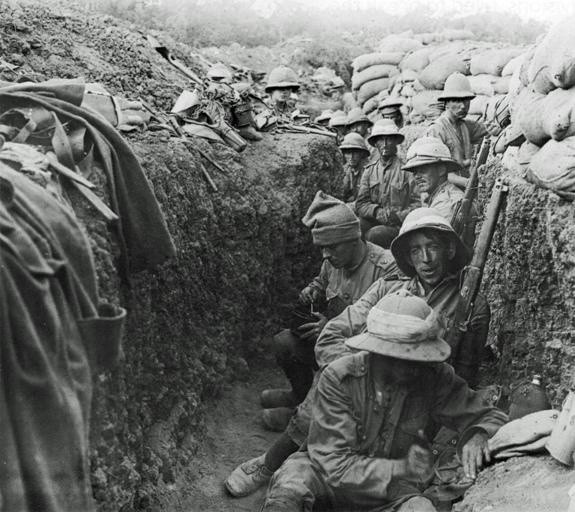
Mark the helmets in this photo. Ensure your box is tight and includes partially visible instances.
[378,98,404,114]
[345,108,374,128]
[318,109,334,124]
[265,67,301,94]
[328,109,348,129]
[437,72,475,102]
[368,119,404,148]
[345,290,450,361]
[400,138,462,173]
[390,207,472,277]
[339,132,371,159]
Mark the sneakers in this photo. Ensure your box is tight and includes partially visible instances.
[226,388,297,498]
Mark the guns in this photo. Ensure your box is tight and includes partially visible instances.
[447,178,510,365]
[449,137,492,234]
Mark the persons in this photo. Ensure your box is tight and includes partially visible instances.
[260,190,396,431]
[253,67,300,114]
[261,288,507,511]
[313,71,501,252]
[224,207,491,496]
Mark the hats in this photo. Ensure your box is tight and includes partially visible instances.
[302,190,361,245]
